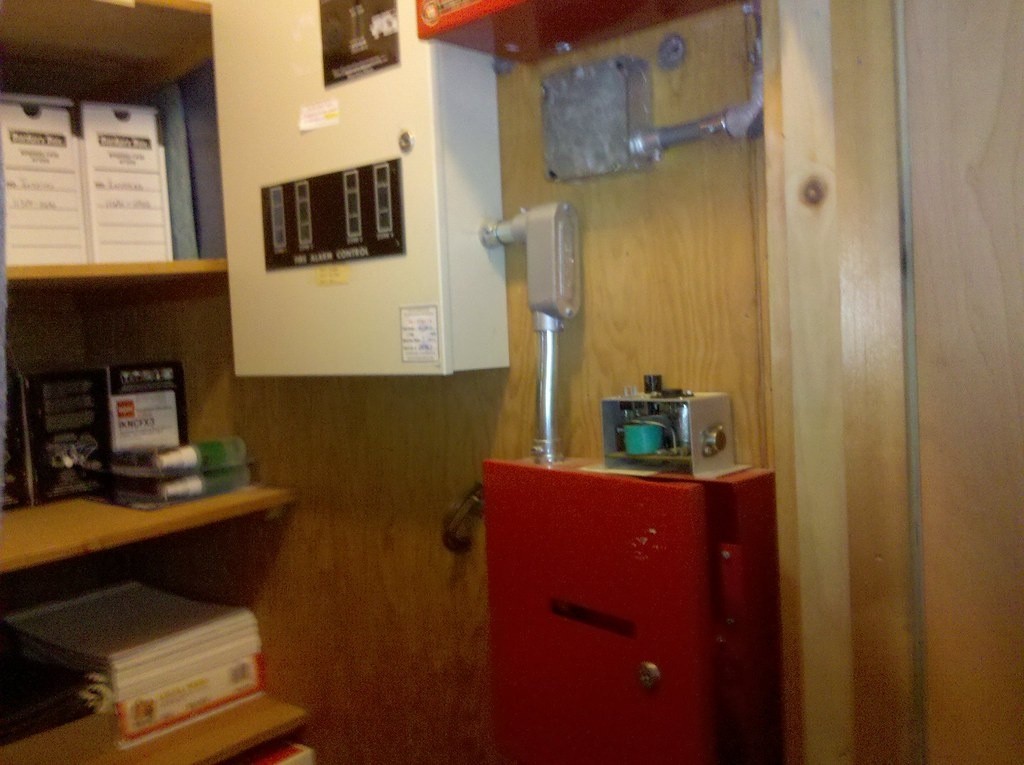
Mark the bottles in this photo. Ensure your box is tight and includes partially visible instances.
[154,436,247,469]
[159,471,251,497]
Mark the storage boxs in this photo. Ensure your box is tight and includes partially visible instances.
[0,378,30,510]
[95,360,189,453]
[23,371,112,505]
[0,94,87,264]
[78,101,174,265]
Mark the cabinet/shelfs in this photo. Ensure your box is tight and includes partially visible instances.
[0,0,312,765]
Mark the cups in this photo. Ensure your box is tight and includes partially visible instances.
[623,423,662,455]
